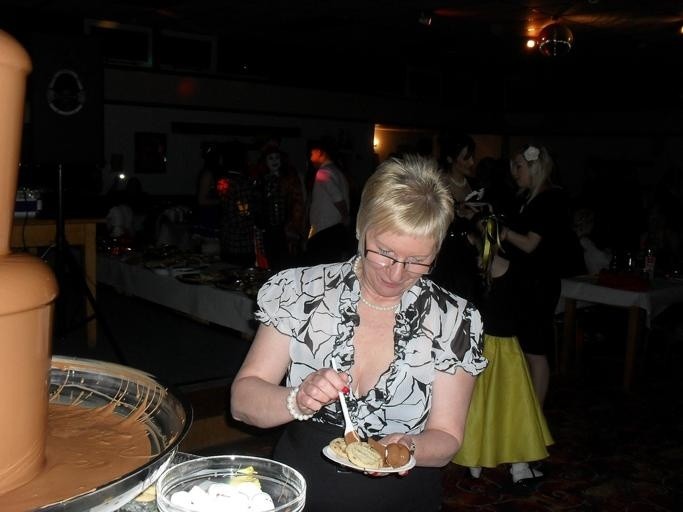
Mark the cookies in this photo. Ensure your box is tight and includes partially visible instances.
[347,441,383,469]
[330,438,348,459]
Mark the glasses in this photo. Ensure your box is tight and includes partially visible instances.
[364,235,436,275]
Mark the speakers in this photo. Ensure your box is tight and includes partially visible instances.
[30,66,104,221]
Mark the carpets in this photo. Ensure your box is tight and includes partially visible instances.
[168,371,682,511]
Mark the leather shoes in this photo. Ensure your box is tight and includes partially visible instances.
[511,464,544,487]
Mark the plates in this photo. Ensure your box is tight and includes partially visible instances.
[320,440,417,476]
[102,232,277,299]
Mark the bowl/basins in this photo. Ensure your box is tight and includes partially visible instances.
[153,453,308,512]
[35,353,191,510]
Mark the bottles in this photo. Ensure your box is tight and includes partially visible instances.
[608,243,659,274]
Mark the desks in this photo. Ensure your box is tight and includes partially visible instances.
[96,243,260,340]
[558,276,683,393]
[10,217,103,351]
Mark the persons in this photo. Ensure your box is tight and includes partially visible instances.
[103,140,349,267]
[229,154,486,511]
[434,134,619,487]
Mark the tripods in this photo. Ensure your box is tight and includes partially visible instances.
[38,222,129,366]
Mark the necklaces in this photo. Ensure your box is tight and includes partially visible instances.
[352,255,401,313]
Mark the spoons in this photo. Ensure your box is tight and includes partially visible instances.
[330,356,360,448]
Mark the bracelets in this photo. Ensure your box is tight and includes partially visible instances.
[410,440,415,455]
[285,387,317,421]
